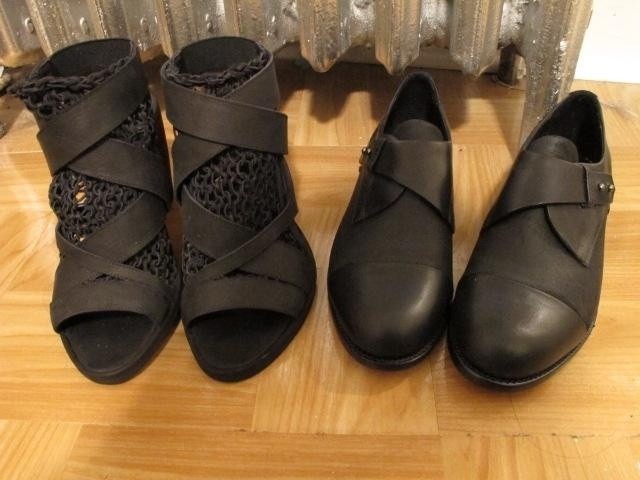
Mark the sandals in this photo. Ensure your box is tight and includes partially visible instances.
[158,35,317,386]
[15,37,182,385]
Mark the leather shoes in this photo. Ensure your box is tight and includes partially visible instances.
[327,70,456,372]
[446,90,615,392]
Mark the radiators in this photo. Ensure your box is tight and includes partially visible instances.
[0,1,593,148]
